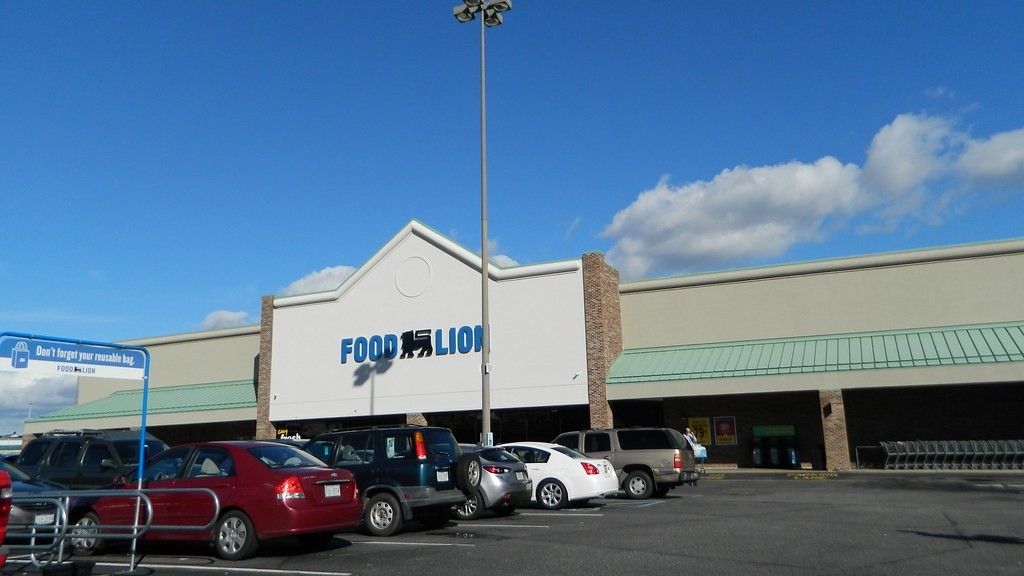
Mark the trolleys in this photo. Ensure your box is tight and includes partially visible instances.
[874,438,1024,472]
[689,443,707,475]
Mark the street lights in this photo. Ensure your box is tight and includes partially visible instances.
[450,0,514,447]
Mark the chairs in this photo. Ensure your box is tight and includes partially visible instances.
[193,456,226,479]
[337,443,369,465]
[522,451,536,463]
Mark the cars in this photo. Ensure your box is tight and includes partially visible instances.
[456,443,534,522]
[285,438,364,465]
[0,453,72,539]
[494,440,620,509]
[66,440,362,562]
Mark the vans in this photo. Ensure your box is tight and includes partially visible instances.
[549,427,702,500]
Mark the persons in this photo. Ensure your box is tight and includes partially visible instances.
[683,428,698,448]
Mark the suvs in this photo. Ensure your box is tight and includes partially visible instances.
[297,421,471,537]
[16,428,227,508]
